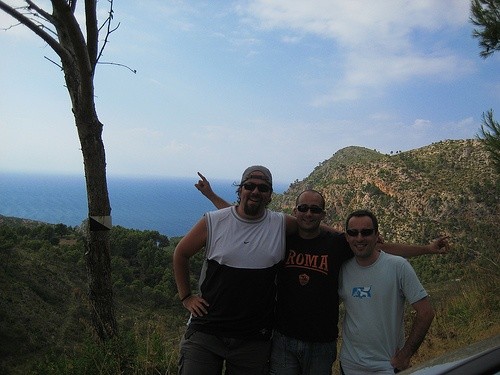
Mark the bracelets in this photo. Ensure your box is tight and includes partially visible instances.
[179,291,192,302]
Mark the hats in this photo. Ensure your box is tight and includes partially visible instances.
[239,165,272,184]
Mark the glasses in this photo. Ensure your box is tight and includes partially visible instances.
[295,204,324,214]
[346,228,377,237]
[241,182,272,192]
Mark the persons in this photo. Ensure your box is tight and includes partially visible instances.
[195,170,449,375]
[339,207,437,375]
[171,167,341,375]
